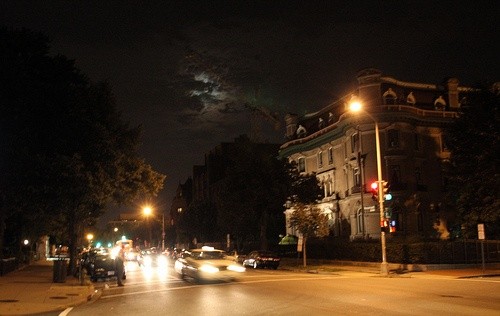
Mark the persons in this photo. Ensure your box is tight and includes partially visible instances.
[116,244,127,286]
[23,244,31,269]
[89,245,100,254]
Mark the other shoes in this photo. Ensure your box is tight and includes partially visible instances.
[118,283,124,286]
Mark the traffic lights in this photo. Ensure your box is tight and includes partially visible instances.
[382,181,392,201]
[370,182,379,202]
[389,220,396,233]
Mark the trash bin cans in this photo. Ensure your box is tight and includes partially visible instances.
[53,259,68,283]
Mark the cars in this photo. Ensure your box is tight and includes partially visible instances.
[226,250,247,263]
[242,250,280,270]
[175,245,245,285]
[84,239,192,283]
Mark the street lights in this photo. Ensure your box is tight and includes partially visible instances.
[348,100,389,274]
[143,207,164,252]
